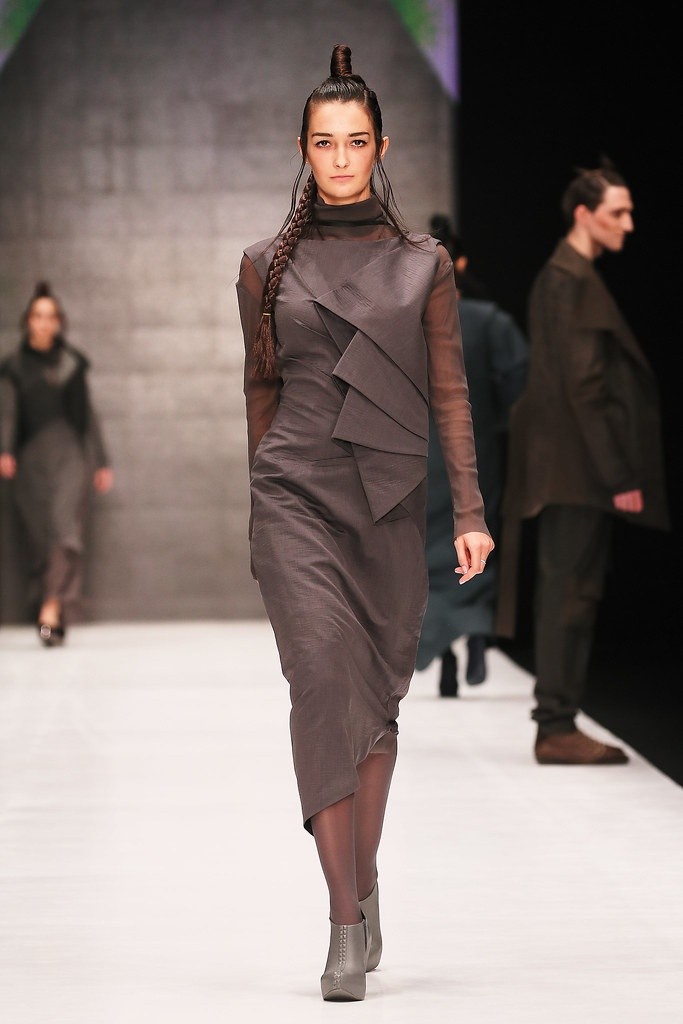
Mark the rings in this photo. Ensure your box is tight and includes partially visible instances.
[481,559,486,564]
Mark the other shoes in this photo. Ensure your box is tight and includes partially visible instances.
[466,639,485,685]
[535,723,628,765]
[38,600,65,646]
[440,654,458,696]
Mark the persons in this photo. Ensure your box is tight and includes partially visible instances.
[0,283,113,644]
[236,43,494,999]
[415,213,526,695]
[504,170,668,766]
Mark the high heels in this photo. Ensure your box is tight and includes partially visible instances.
[320,917,366,1000]
[359,882,383,971]
